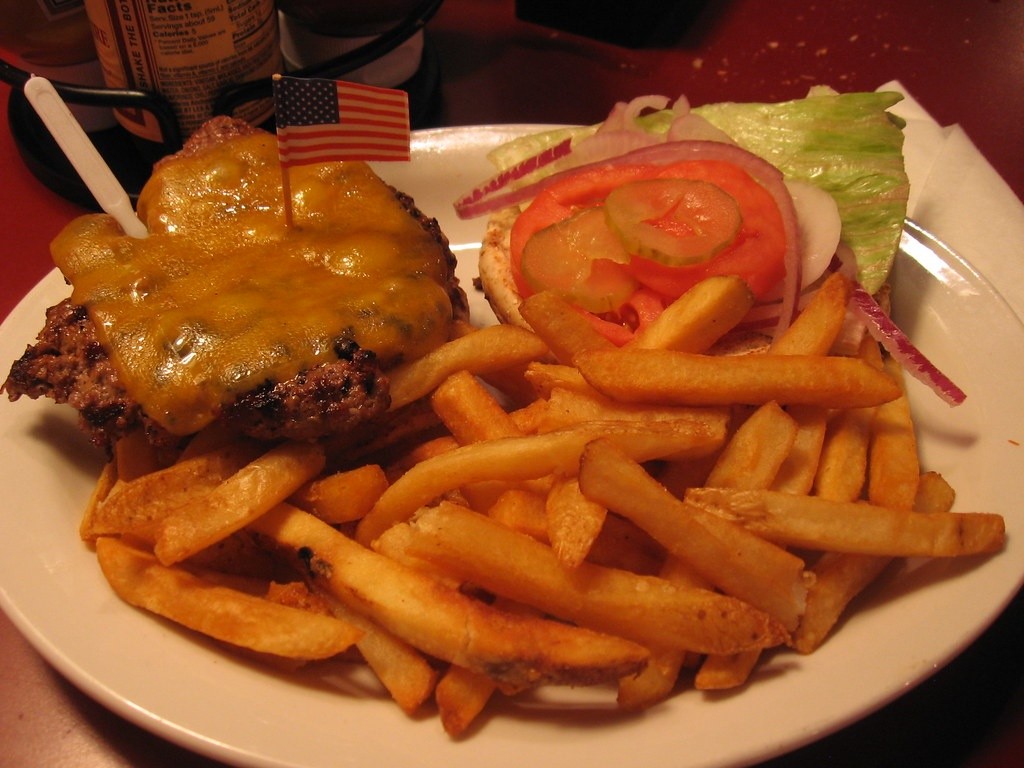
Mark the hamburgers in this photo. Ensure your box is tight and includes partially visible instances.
[0,85,970,445]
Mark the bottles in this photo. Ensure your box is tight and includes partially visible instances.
[83,0,285,147]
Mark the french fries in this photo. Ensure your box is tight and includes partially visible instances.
[80,278,1006,733]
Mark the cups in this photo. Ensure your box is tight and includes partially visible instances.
[16,57,119,133]
[277,4,424,88]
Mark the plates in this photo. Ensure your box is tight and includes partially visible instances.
[0,126,1023,768]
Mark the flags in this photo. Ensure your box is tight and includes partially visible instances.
[274,76,412,169]
